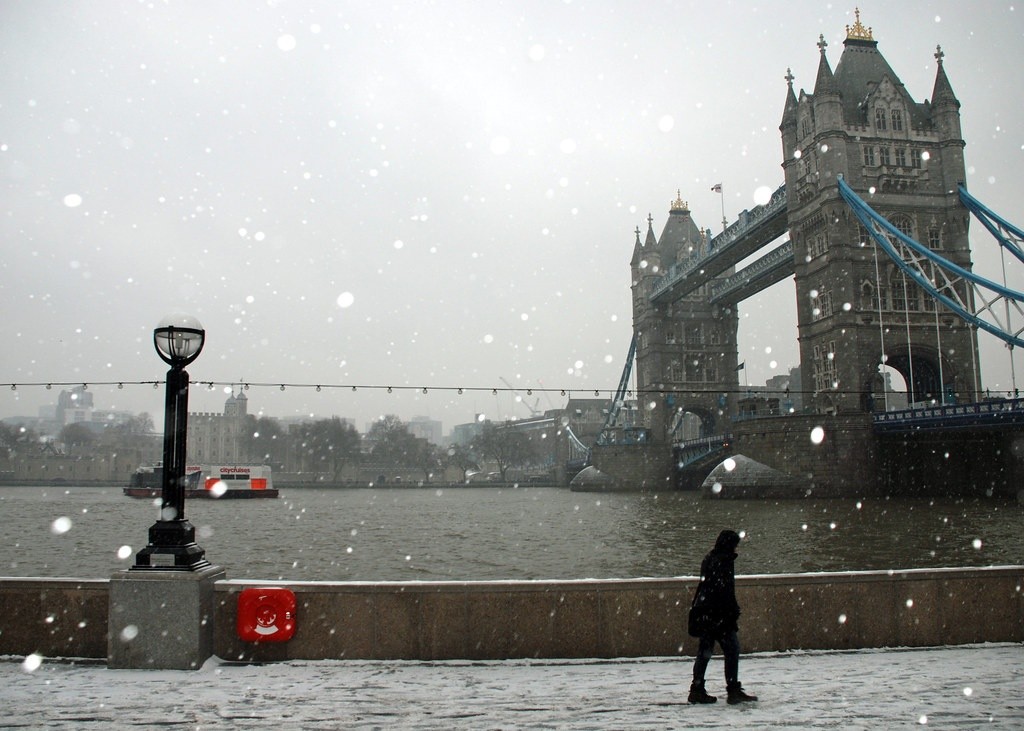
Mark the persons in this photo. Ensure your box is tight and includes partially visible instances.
[688,530,758,705]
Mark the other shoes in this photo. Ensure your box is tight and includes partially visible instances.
[687,680,717,704]
[726,682,758,705]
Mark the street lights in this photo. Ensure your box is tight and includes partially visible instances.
[129,314,212,572]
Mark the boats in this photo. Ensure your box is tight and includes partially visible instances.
[123,461,279,500]
